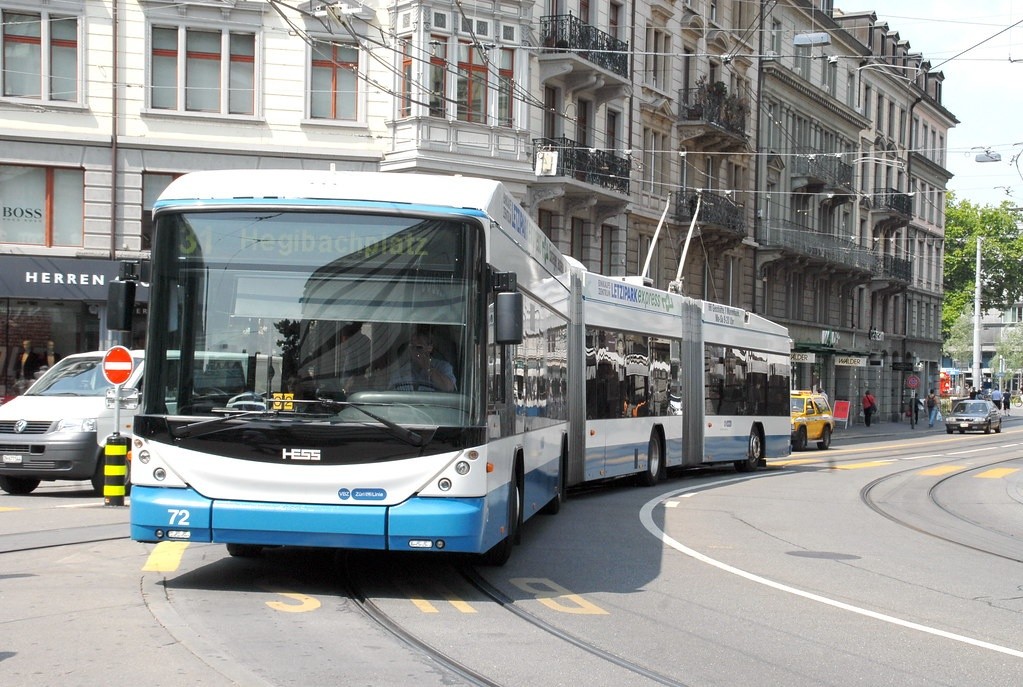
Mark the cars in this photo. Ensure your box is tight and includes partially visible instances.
[790,389,835,452]
[945,399,1003,433]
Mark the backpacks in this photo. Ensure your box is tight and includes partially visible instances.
[927,395,936,408]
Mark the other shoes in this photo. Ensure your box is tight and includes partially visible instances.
[1008,414,1010,417]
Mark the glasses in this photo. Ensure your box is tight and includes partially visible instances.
[408,343,429,352]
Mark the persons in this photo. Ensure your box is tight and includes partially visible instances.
[976,389,982,400]
[909,393,924,424]
[1003,389,1010,416]
[338,328,369,387]
[811,388,829,402]
[1018,384,1023,403]
[40,341,61,370]
[13,339,40,396]
[981,386,988,396]
[862,390,874,427]
[388,333,457,392]
[970,387,977,399]
[991,386,1001,410]
[926,389,938,428]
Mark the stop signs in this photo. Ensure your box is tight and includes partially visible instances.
[102,345,134,386]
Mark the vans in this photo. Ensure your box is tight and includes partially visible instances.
[0,348,283,498]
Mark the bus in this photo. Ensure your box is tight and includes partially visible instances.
[128,169,794,569]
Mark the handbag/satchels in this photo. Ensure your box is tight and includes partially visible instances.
[936,411,943,422]
[870,404,878,412]
[906,407,912,416]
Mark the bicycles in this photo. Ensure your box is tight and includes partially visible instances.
[1010,394,1022,408]
